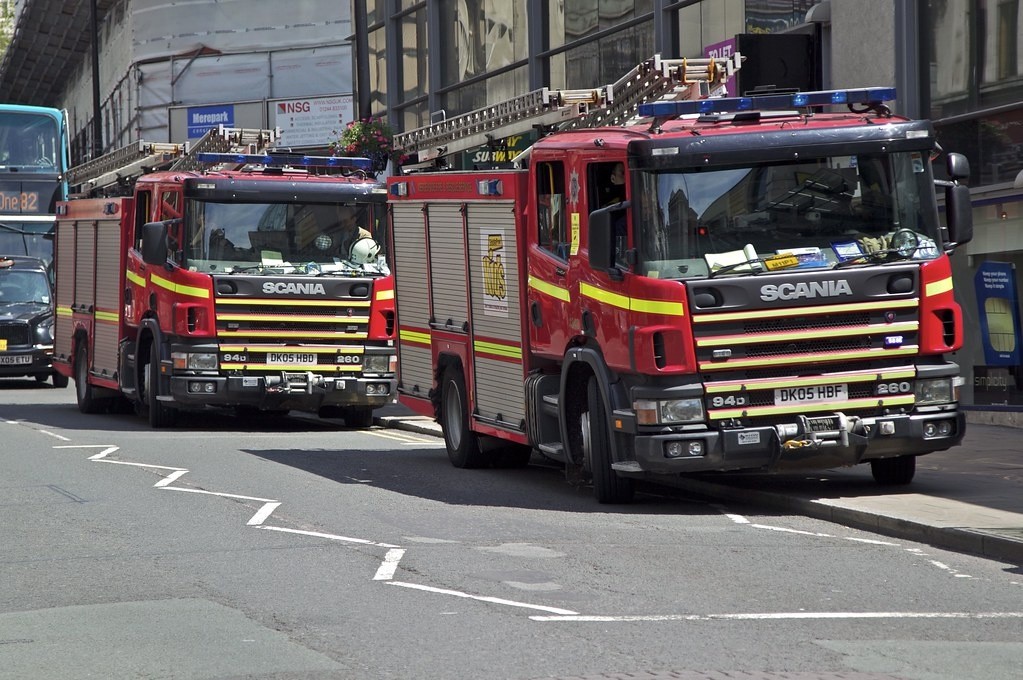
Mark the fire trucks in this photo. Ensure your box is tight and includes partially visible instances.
[386,50,973,506]
[52,122,400,429]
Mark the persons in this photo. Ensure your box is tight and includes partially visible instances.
[190,205,222,245]
[338,206,373,257]
[605,162,626,235]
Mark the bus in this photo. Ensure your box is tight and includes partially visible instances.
[0,104,79,305]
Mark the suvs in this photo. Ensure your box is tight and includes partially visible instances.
[0,255,68,388]
